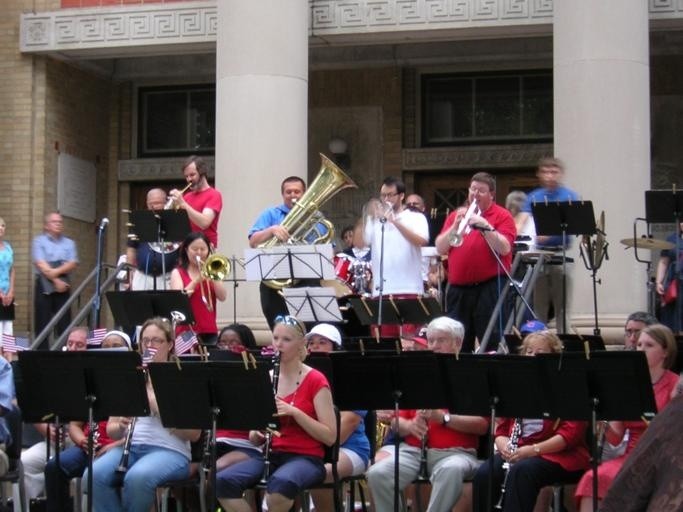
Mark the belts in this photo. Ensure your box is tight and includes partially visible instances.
[538,247,562,251]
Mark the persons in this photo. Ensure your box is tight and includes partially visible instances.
[247,176,444,333]
[0,219,16,344]
[657,231,682,332]
[0,315,369,512]
[128,157,229,346]
[365,310,682,512]
[434,158,579,351]
[32,212,80,350]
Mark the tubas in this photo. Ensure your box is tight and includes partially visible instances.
[260,153,360,288]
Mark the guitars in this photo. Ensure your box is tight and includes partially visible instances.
[659,263,682,308]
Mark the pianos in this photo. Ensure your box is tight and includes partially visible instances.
[511,251,573,266]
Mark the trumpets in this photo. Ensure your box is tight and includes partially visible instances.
[446,200,482,248]
[163,183,197,214]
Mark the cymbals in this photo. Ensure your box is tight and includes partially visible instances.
[620,237,675,251]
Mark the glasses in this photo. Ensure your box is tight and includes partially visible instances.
[276,316,305,337]
[141,337,166,345]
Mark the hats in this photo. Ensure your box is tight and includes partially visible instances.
[412,327,427,346]
[306,323,341,346]
[521,320,544,333]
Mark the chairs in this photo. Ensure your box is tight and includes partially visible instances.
[0,405,597,511]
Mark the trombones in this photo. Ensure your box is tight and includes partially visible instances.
[197,254,231,311]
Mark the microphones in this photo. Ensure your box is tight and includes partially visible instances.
[98,217,109,230]
[379,217,386,223]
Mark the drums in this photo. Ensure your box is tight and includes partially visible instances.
[319,253,363,300]
[136,240,181,276]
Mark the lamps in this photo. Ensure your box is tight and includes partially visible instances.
[329,138,349,172]
